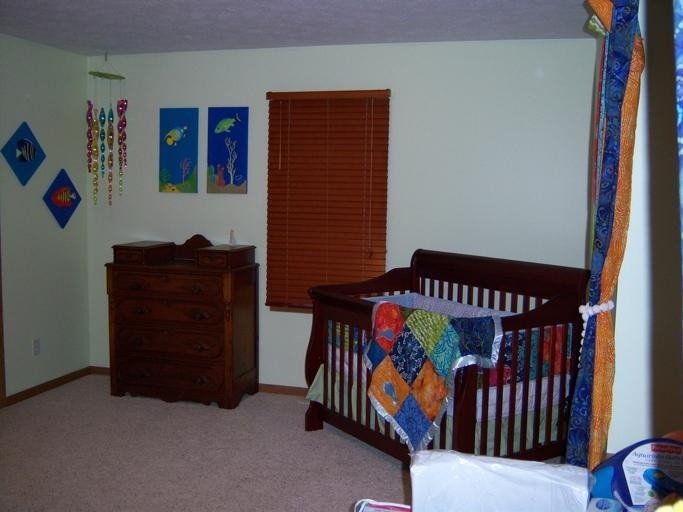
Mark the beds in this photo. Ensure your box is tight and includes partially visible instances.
[303,249,592,461]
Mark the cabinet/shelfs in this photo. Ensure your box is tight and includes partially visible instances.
[104,235,259,411]
